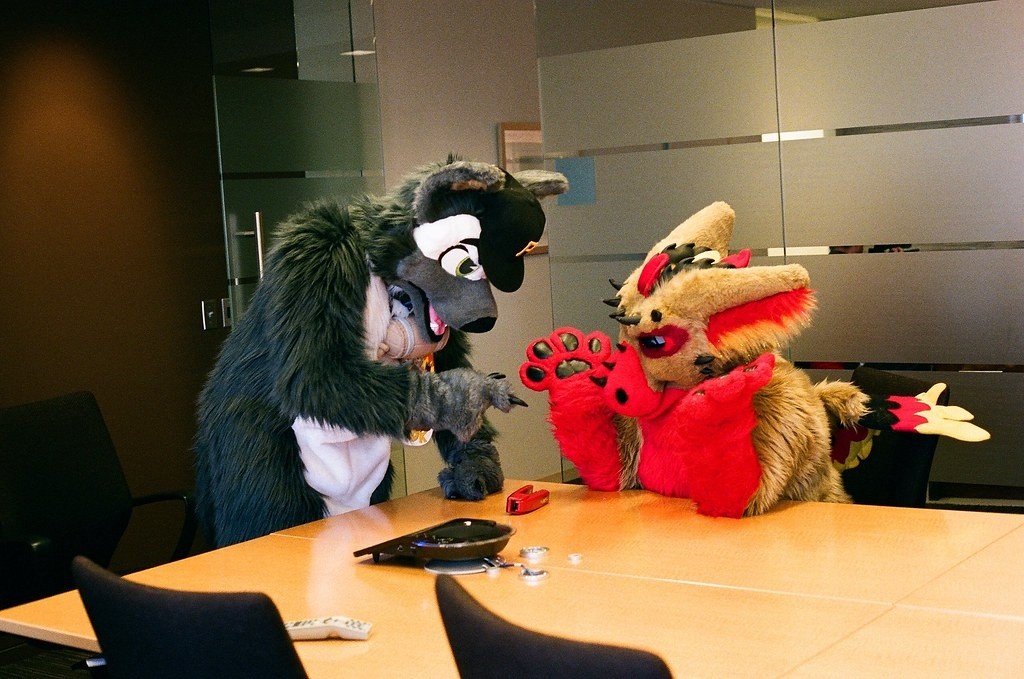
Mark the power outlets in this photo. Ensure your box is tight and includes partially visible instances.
[221,297,233,328]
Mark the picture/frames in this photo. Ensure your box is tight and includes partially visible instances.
[498,122,585,255]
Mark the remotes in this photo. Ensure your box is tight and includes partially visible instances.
[284,616,372,640]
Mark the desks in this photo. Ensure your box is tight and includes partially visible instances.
[0,475,1024,679]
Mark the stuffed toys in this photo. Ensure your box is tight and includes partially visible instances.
[195,155,568,548]
[519,200,991,519]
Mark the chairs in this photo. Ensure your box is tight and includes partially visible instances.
[71,553,309,679]
[842,363,950,507]
[0,391,198,654]
[434,573,675,679]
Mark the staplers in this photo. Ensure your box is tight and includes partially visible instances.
[506,484,549,514]
